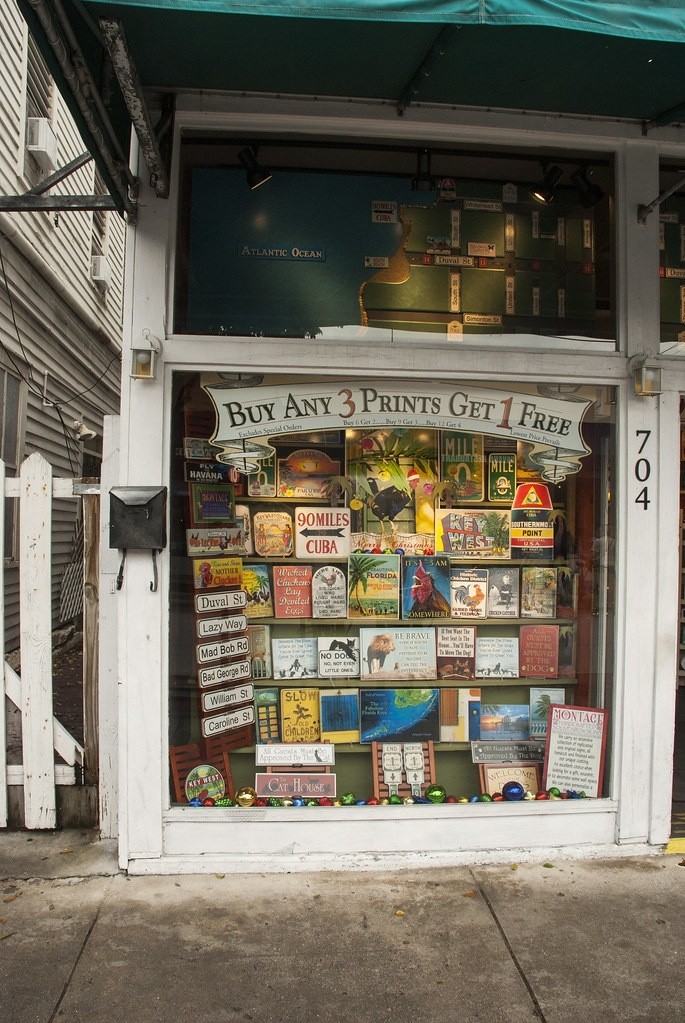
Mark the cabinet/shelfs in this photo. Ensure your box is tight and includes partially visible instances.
[227,440,580,756]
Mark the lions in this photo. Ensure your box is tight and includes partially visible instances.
[363,634,395,674]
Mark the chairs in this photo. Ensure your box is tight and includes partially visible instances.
[266,738,331,773]
[169,734,235,803]
[371,740,437,799]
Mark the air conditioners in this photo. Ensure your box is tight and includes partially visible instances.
[26,115,59,170]
[91,255,111,291]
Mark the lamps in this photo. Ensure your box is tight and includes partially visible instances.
[527,163,565,206]
[128,327,163,382]
[411,151,438,192]
[237,148,273,191]
[626,352,665,398]
[73,420,97,442]
[569,167,605,211]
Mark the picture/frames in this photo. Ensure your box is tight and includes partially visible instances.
[483,762,541,796]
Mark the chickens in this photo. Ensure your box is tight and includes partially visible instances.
[411,560,451,615]
